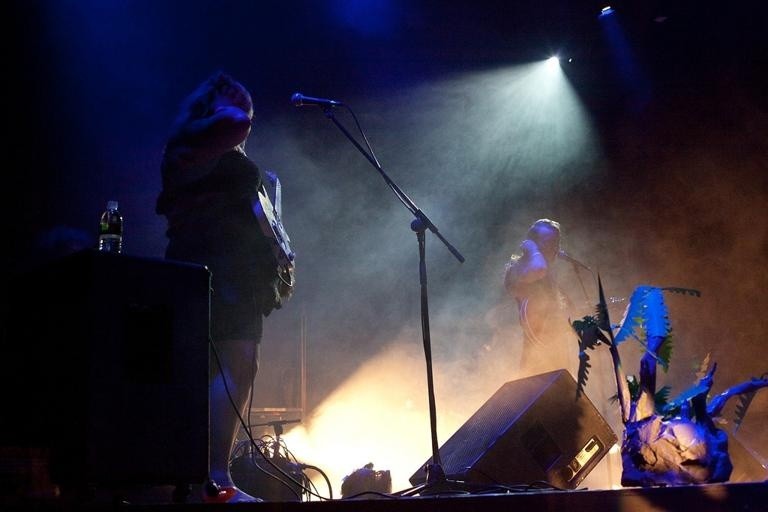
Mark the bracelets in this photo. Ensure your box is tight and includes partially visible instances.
[524,248,543,260]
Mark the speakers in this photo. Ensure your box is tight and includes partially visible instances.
[409,368,618,492]
[1,244,219,500]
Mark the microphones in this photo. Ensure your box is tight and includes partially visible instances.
[291,93,342,108]
[558,251,591,271]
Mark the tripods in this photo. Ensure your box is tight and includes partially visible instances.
[325,112,510,498]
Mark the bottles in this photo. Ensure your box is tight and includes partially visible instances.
[97,200,124,254]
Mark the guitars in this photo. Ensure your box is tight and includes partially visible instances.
[519,285,628,350]
[250,171,296,269]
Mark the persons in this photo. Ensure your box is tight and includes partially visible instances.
[153,76,296,503]
[502,217,578,380]
